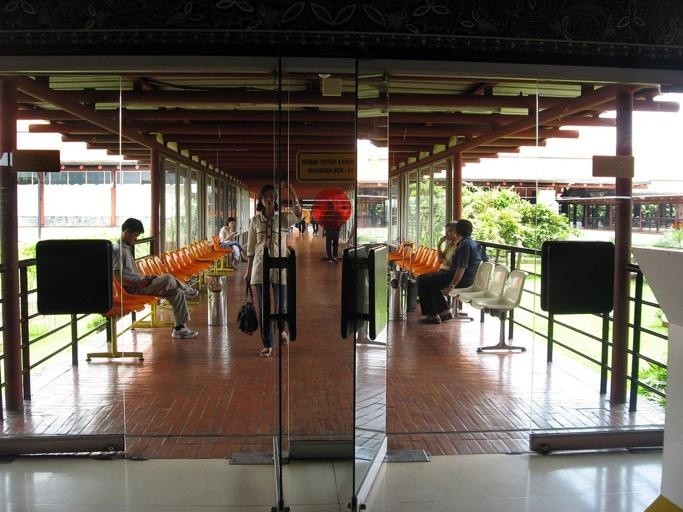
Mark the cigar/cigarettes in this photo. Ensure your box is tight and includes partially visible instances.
[440,235,446,237]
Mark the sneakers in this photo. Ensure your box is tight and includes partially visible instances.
[184,288,198,298]
[172,327,198,338]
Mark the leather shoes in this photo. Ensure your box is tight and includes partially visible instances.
[419,313,454,324]
[282,331,288,347]
[259,348,272,357]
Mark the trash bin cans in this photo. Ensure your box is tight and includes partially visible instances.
[207,275,227,326]
[390,270,408,321]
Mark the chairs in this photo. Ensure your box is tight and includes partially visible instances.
[86,235,236,364]
[377,240,529,353]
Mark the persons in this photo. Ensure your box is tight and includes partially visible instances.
[243,180,305,356]
[435,220,459,272]
[297,218,305,235]
[416,219,489,323]
[319,202,342,263]
[218,218,247,261]
[311,212,319,235]
[112,218,200,339]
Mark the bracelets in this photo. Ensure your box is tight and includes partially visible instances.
[290,197,299,208]
[448,281,456,288]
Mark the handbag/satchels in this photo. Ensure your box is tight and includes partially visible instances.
[237,302,258,336]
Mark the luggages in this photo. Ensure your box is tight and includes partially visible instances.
[402,244,417,312]
